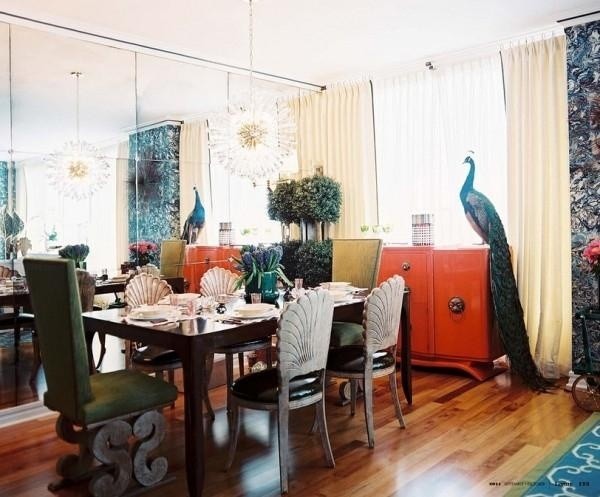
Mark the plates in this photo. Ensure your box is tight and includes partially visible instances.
[127,306,181,323]
[320,281,354,303]
[231,303,278,319]
[164,292,201,302]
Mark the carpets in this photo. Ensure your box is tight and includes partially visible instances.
[501,410,600,496]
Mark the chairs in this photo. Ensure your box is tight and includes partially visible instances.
[20,236,405,496]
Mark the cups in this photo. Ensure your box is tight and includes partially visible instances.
[251,293,262,305]
[188,301,197,317]
[295,279,303,290]
[170,294,179,310]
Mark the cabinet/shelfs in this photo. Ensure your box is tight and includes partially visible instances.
[376,245,505,380]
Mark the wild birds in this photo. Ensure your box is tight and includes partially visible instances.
[179,185,207,243]
[457,148,552,393]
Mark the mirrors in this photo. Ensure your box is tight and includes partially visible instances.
[0,20,299,411]
